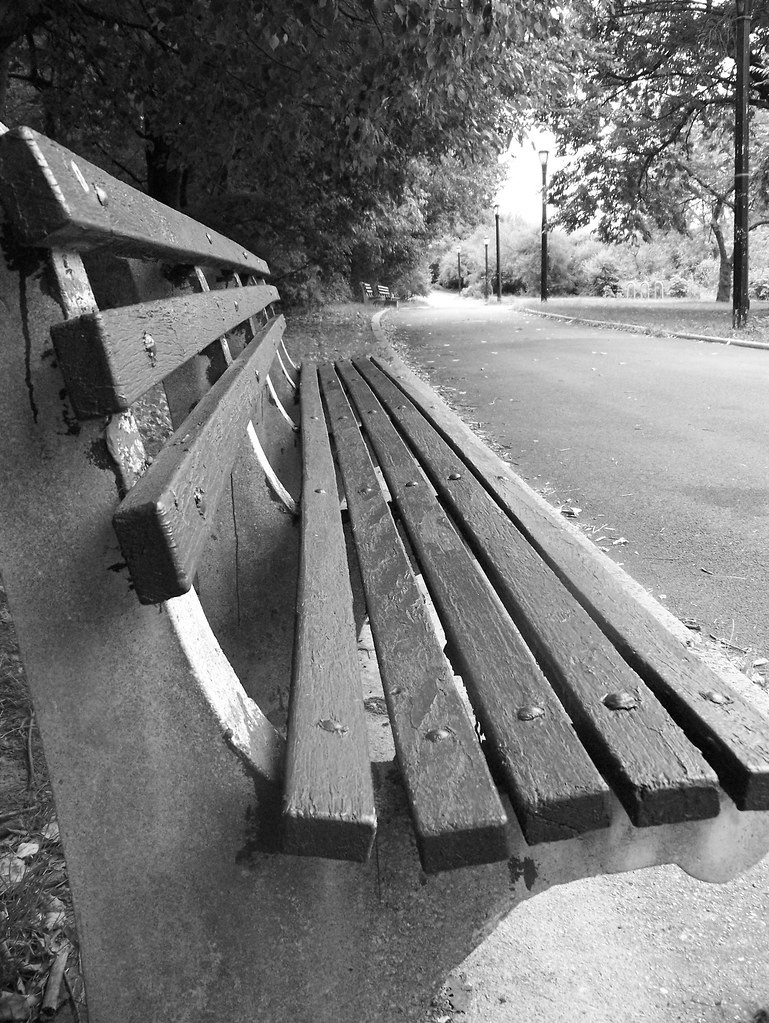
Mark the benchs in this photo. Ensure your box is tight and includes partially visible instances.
[0,119,767,1022]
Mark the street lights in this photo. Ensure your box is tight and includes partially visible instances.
[539,150,549,302]
[494,204,503,302]
[456,245,463,294]
[483,236,491,298]
